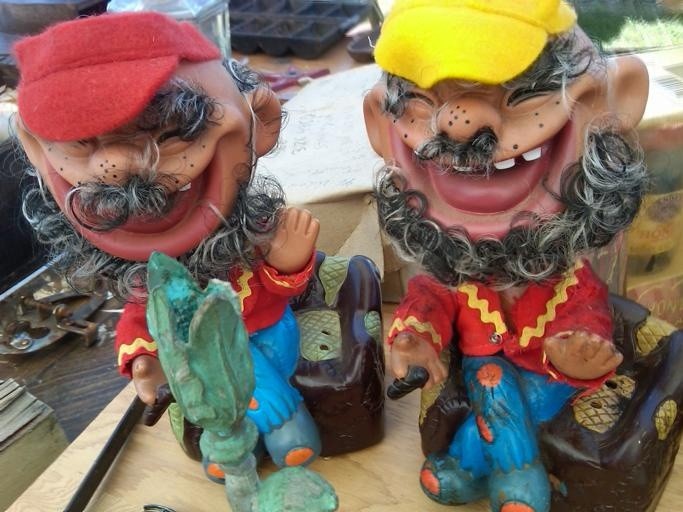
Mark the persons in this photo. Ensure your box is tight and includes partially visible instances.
[364,1,683,512]
[8,10,388,486]
